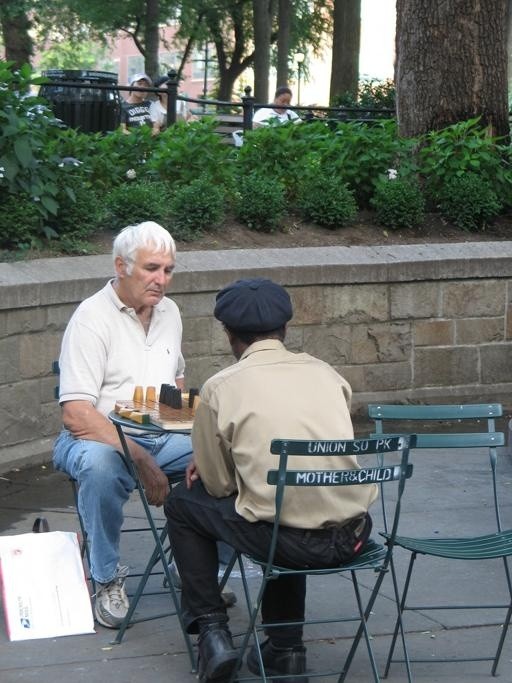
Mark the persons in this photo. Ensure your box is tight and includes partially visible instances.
[252,87,302,129]
[119,72,152,134]
[51,220,238,628]
[163,276,374,681]
[150,76,198,138]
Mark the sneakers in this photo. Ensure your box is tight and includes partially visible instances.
[164,559,238,607]
[91,562,132,630]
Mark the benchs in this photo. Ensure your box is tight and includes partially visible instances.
[196,111,254,149]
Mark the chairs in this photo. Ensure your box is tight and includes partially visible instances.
[223,430,420,683]
[50,355,223,629]
[365,399,512,682]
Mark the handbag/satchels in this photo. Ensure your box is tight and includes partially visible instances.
[0,518,97,642]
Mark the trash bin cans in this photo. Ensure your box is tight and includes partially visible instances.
[37,69,121,133]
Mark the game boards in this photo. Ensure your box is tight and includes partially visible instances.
[113,393,201,431]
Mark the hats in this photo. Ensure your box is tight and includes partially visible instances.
[128,73,152,86]
[213,278,294,334]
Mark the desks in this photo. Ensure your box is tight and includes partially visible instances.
[106,391,270,674]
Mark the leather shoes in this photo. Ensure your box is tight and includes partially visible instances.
[196,622,242,683]
[245,636,308,683]
